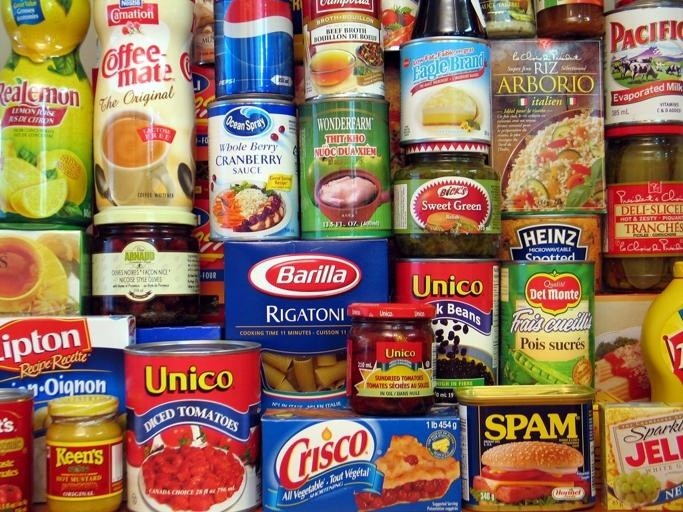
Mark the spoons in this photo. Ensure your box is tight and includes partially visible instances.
[94,163,118,207]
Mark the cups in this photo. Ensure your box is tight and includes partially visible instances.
[98,108,176,207]
[0,235,45,314]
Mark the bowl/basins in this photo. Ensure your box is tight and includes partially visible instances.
[314,167,382,228]
[309,48,356,86]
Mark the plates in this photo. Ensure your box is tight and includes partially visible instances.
[311,75,356,95]
[137,444,247,512]
[211,178,292,239]
[0,235,68,318]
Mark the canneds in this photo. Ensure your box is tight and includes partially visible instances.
[0,0,683,512]
